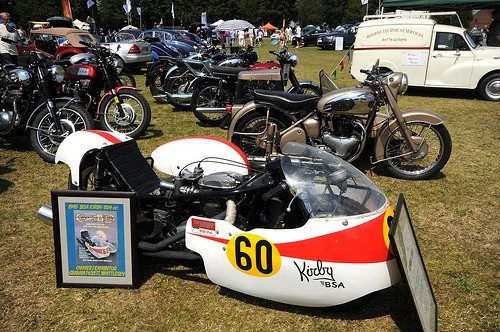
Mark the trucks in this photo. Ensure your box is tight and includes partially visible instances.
[350,11,500,102]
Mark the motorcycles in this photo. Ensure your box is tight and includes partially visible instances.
[76,229,118,258]
[144,41,259,112]
[0,21,151,163]
[36,130,403,308]
[177,44,323,125]
[228,58,453,180]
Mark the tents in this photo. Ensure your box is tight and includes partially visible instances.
[381,0,500,13]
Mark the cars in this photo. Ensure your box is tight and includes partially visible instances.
[30,17,216,77]
[316,23,360,49]
[270,30,282,41]
[292,29,324,48]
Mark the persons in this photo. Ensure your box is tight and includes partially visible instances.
[189,21,303,49]
[0,12,26,66]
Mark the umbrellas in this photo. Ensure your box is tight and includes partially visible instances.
[209,19,279,31]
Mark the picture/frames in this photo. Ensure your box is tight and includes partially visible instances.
[388,193,438,332]
[51,190,139,289]
